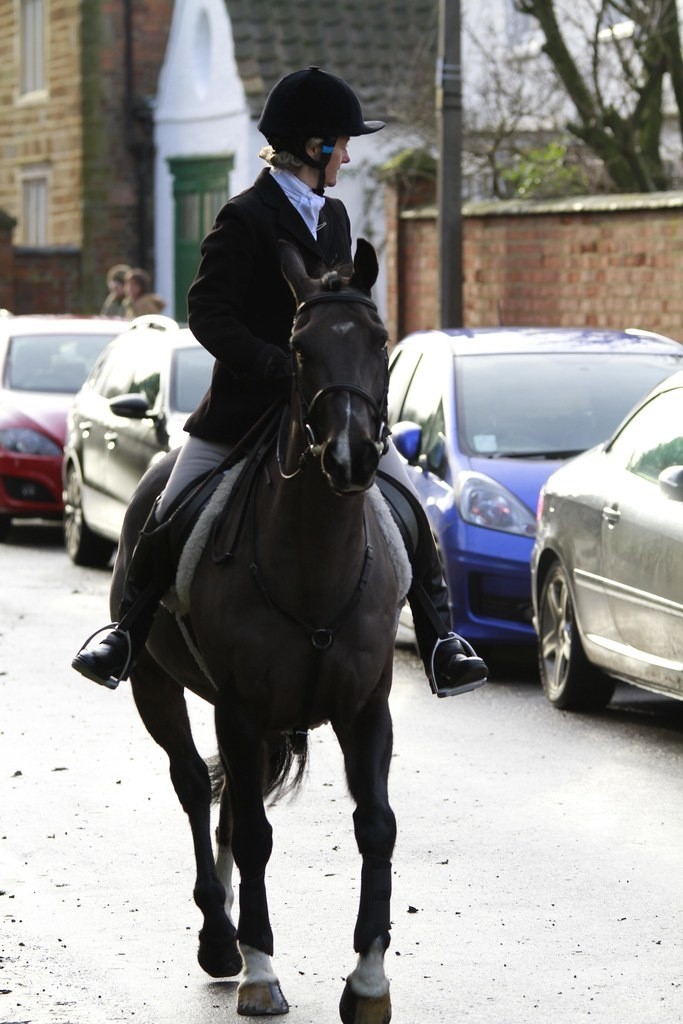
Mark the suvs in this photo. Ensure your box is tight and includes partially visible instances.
[60,315,213,565]
[0,308,135,549]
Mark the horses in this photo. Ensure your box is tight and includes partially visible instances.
[108,236,411,1023]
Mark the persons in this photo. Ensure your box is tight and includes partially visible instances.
[122,268,165,317]
[69,66,488,695]
[100,264,132,315]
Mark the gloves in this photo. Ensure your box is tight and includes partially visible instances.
[250,344,294,400]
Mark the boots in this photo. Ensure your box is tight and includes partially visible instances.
[406,531,488,694]
[71,496,207,685]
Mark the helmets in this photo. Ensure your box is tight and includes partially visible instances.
[256,66,386,165]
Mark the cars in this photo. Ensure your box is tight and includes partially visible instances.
[387,325,680,689]
[531,368,682,713]
